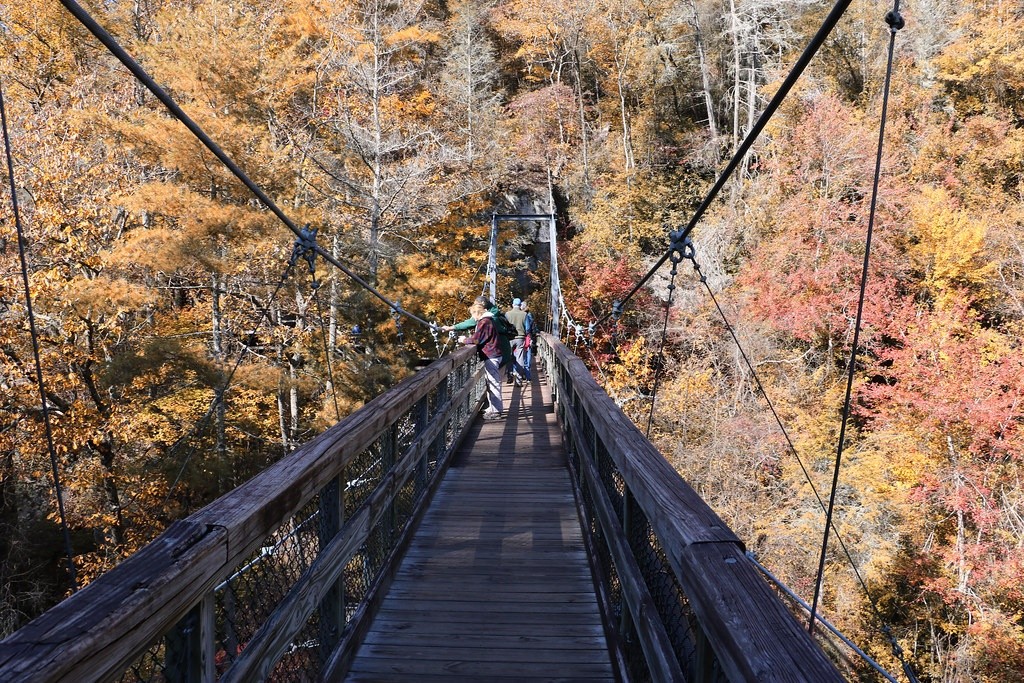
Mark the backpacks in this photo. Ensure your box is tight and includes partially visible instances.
[496,314,518,340]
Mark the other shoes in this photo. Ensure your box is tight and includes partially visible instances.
[526,378,531,383]
[506,375,514,384]
[482,412,502,420]
[516,382,523,387]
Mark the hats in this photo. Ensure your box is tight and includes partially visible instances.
[513,298,522,304]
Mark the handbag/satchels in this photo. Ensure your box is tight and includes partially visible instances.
[525,335,531,349]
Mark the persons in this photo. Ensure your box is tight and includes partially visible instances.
[504,298,538,388]
[458,301,504,420]
[441,297,518,376]
